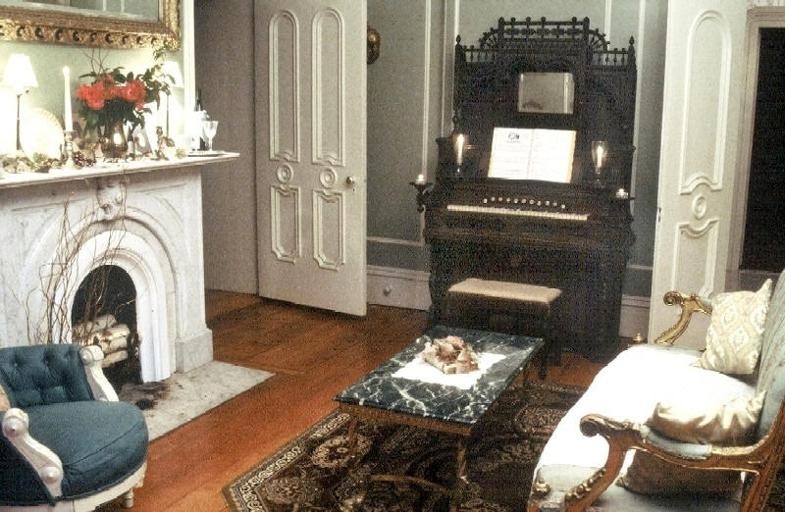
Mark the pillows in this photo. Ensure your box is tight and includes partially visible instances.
[690,277,774,379]
[616,388,770,501]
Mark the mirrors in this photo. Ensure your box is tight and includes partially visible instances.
[0,0,183,49]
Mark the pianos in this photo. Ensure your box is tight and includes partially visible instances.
[423,137,638,363]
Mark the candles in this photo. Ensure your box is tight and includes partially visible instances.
[416,173,425,183]
[456,132,465,166]
[595,142,605,168]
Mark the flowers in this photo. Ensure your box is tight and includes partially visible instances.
[72,51,178,130]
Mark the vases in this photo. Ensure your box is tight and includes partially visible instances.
[100,121,129,163]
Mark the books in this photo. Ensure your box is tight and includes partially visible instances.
[486,124,577,186]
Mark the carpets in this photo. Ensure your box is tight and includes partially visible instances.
[219,380,785,512]
[136,360,277,446]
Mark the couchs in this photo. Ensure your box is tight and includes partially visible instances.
[527,268,785,512]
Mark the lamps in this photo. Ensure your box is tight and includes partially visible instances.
[2,51,39,152]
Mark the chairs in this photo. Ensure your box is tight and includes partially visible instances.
[0,342,151,511]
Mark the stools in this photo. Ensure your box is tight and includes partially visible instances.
[443,276,565,380]
